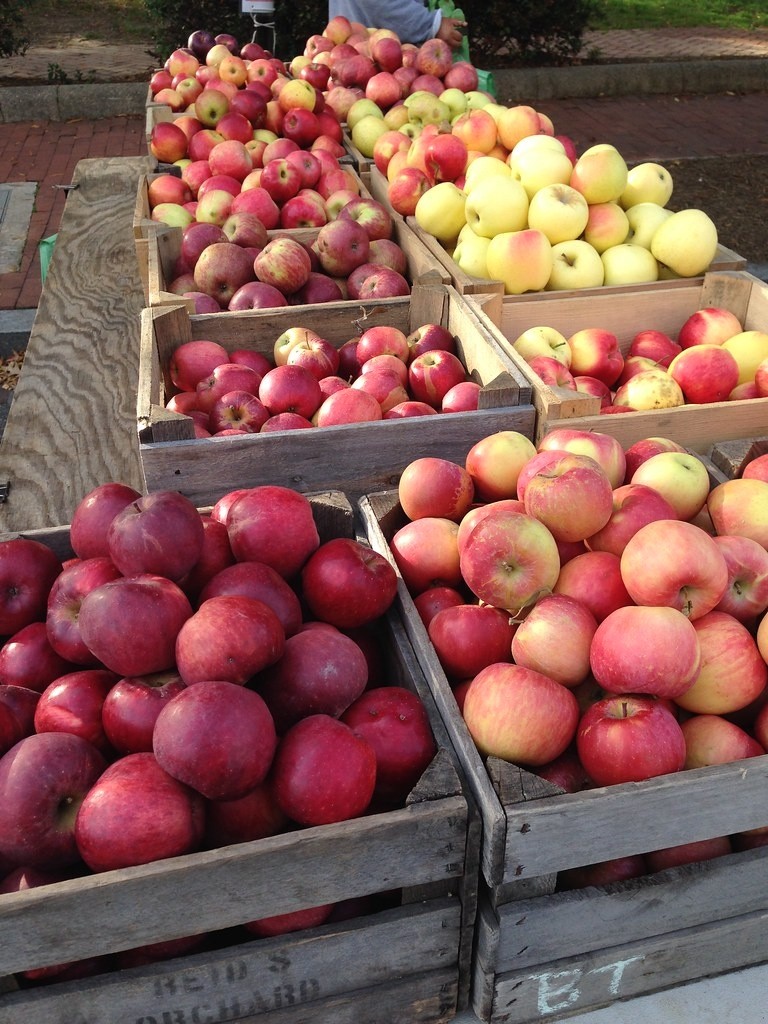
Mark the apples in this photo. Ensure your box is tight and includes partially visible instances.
[147,14,719,315]
[0,484,432,990]
[512,308,768,416]
[392,425,768,888]
[162,320,484,439]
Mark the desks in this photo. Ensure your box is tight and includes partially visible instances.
[0,156,146,533]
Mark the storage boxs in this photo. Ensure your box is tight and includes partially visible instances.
[0,121,767,1024]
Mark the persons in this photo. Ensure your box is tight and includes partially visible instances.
[329,0,468,51]
